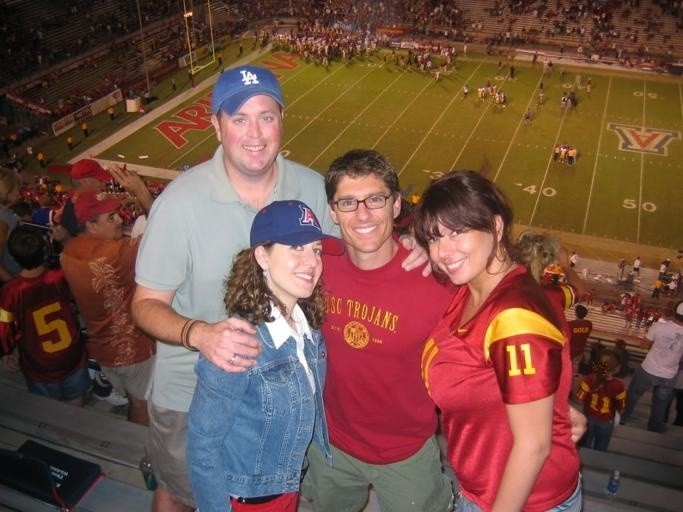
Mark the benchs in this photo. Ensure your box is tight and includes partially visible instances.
[0,295,683,509]
[206,1,682,61]
[0,0,231,162]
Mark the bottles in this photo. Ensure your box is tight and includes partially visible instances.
[602,468,622,497]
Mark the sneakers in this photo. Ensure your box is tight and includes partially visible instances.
[91,388,129,407]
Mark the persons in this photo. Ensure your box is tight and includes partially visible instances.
[2,2,682,123]
[296,148,586,511]
[187,199,333,512]
[515,231,682,450]
[2,160,166,423]
[2,88,149,173]
[129,67,433,511]
[554,142,577,163]
[403,169,584,511]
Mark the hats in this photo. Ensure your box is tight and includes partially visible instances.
[675,302,683,315]
[210,65,285,121]
[72,187,127,224]
[249,200,346,255]
[69,158,113,183]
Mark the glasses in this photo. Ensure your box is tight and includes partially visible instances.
[333,191,394,213]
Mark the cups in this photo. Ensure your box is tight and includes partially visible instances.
[138,454,158,491]
